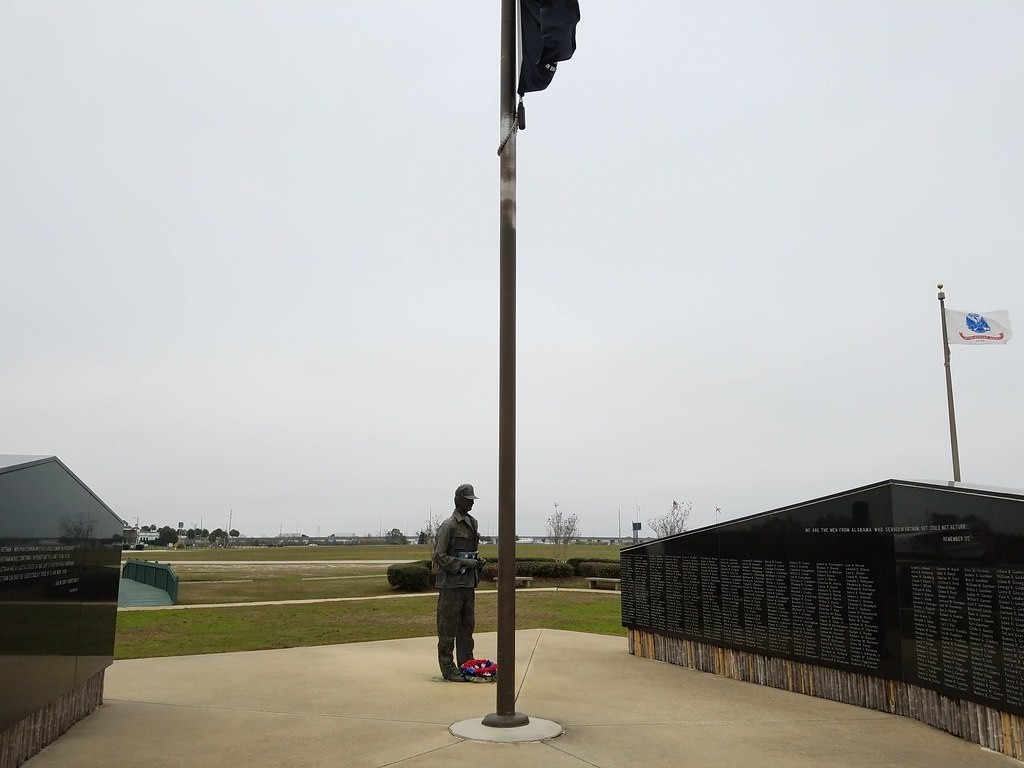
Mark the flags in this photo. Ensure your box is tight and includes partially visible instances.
[944,308,1012,345]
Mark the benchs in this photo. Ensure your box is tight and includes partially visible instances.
[585,577,621,591]
[493,577,533,588]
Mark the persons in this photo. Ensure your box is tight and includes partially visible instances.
[433,484,488,682]
